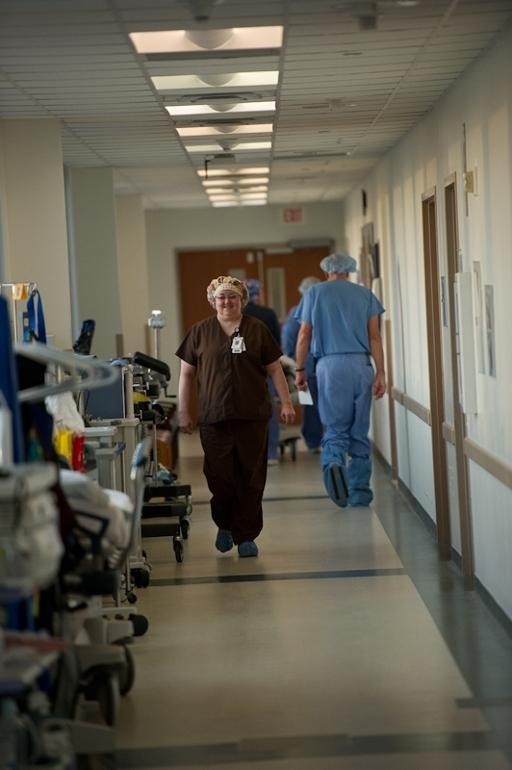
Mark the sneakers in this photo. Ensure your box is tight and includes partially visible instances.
[307,446,319,453]
[238,541,258,556]
[348,487,374,506]
[215,528,233,552]
[266,459,279,465]
[326,463,348,508]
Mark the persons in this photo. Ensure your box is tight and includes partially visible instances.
[291,249,388,509]
[239,278,286,466]
[175,276,297,559]
[277,275,325,454]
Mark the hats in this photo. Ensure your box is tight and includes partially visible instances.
[244,279,264,303]
[206,276,250,309]
[320,253,356,273]
[298,277,321,294]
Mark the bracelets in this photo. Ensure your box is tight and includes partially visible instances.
[295,367,306,372]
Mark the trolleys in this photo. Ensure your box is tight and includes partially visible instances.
[270,342,311,467]
